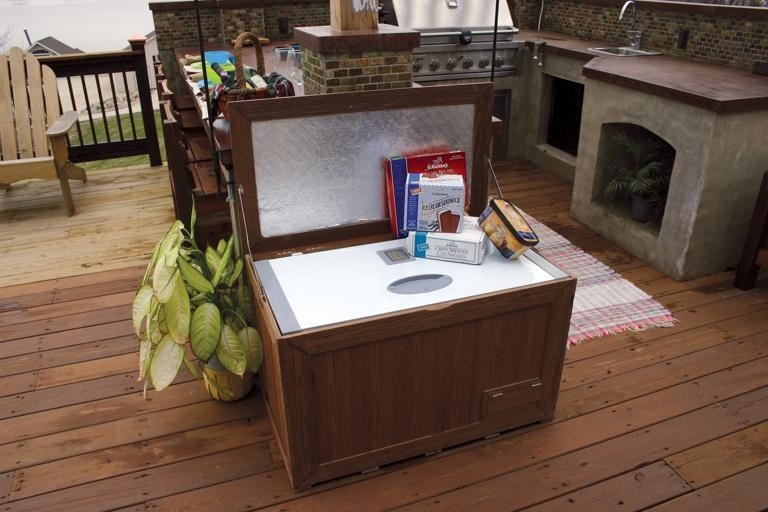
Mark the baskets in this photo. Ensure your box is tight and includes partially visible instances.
[216,33,270,122]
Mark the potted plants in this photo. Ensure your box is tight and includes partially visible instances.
[600,130,684,225]
[130,188,266,405]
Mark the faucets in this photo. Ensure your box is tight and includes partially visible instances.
[618,0,643,50]
[537,0,545,32]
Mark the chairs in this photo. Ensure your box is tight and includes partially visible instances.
[0,46,87,219]
[152,50,242,258]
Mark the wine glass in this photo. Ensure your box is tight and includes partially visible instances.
[273,47,305,96]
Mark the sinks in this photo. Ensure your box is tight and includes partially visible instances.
[532,33,568,42]
[584,45,664,59]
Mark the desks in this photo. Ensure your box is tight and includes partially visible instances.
[172,34,505,225]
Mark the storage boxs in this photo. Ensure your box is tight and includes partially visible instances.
[225,82,581,496]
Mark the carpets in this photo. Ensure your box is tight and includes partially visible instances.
[508,202,682,352]
